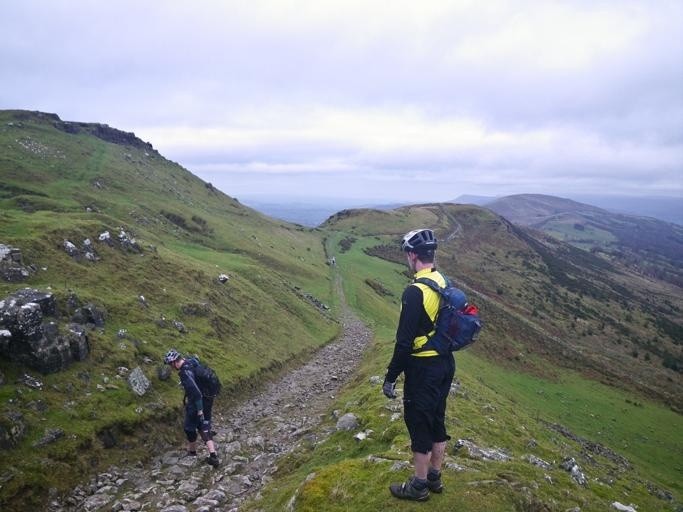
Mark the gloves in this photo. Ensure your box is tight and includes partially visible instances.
[381,373,398,399]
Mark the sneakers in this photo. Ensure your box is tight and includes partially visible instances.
[183,447,196,457]
[389,480,429,502]
[408,476,442,494]
[204,457,219,466]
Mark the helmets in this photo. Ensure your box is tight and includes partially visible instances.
[163,350,181,365]
[400,228,437,254]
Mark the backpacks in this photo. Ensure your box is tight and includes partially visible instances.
[181,359,220,406]
[409,272,481,355]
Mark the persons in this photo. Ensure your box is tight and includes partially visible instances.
[165,348,220,469]
[382,228,455,502]
